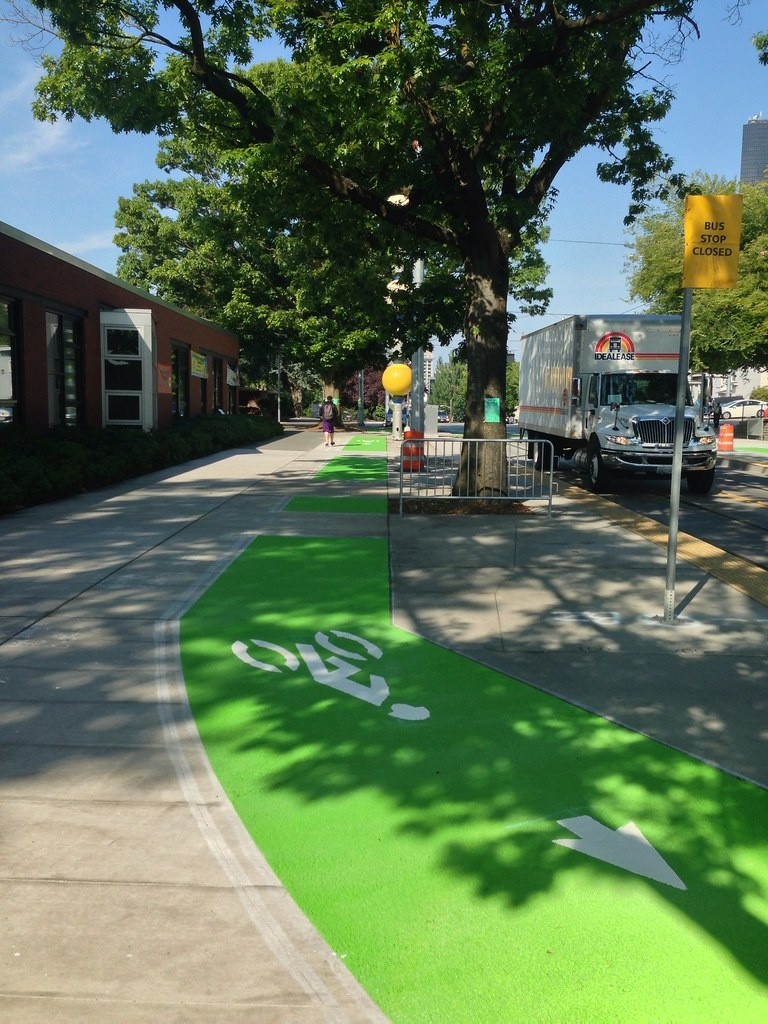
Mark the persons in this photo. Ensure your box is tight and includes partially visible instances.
[319,396,338,447]
[713,402,722,430]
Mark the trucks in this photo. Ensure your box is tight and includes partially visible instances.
[514,315,719,496]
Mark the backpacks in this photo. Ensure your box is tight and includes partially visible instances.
[323,402,334,420]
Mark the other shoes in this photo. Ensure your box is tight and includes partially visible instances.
[331,442,335,445]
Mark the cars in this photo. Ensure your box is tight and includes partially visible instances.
[438,411,450,423]
[386,408,407,427]
[710,399,768,419]
